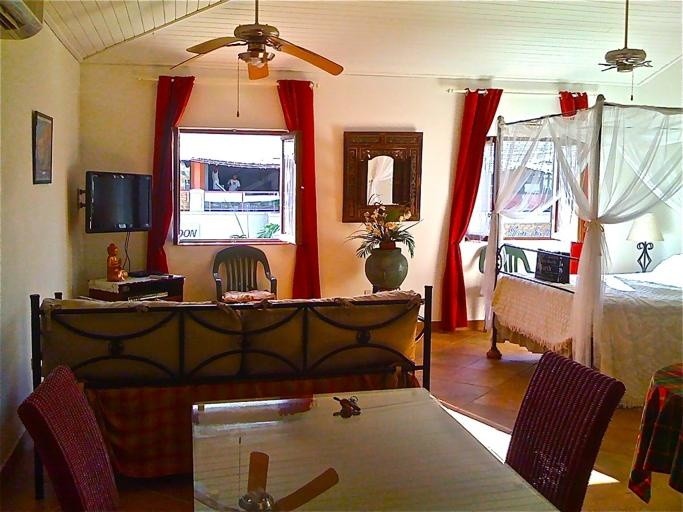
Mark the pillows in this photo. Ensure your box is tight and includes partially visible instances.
[652,252,683,286]
[243,287,421,379]
[40,298,243,384]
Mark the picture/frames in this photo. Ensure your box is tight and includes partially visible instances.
[31,110,53,186]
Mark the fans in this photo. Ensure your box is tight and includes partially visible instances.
[194,451,339,510]
[170,0,344,80]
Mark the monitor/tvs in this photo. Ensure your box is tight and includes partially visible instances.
[86,171,152,232]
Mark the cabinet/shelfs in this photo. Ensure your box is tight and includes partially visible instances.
[87,271,186,301]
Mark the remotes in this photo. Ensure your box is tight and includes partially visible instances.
[153,272,173,277]
[150,275,168,280]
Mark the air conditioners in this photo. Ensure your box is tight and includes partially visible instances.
[0,0,45,42]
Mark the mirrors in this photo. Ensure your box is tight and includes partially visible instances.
[343,131,421,224]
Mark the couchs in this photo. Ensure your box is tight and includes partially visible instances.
[29,286,433,490]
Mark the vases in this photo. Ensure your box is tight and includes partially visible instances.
[364,247,409,293]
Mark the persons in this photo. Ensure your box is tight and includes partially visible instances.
[209,166,221,191]
[105,242,127,282]
[226,172,242,191]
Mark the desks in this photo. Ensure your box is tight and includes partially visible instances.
[628,363,683,503]
[190,387,561,512]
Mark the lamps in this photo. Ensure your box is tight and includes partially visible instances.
[238,39,276,68]
[238,487,274,511]
[625,211,664,272]
[598,0,653,72]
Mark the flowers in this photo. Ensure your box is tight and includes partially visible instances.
[342,202,416,262]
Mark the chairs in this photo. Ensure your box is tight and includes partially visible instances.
[17,364,124,512]
[504,348,627,511]
[212,246,277,304]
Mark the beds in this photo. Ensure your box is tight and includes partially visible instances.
[486,95,683,410]
